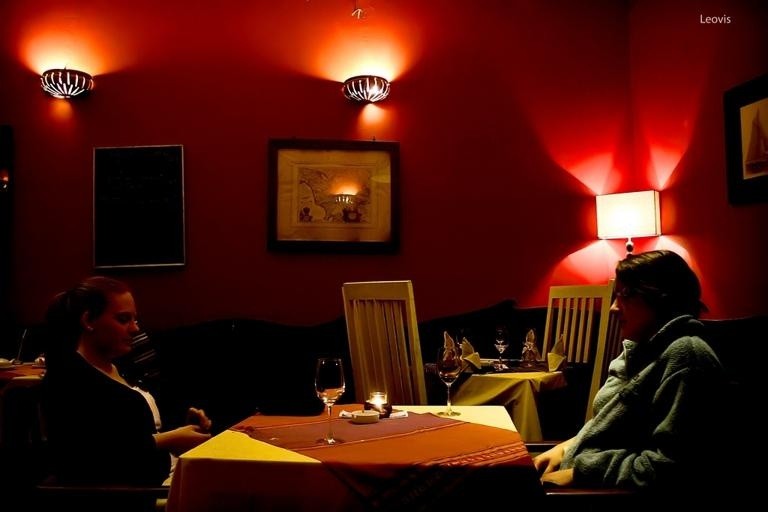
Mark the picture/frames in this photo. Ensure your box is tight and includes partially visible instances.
[268,136,402,258]
[721,75,768,209]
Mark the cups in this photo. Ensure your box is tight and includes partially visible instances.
[369,392,388,412]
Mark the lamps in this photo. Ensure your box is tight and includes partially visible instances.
[595,187,661,257]
[40,68,95,101]
[341,75,390,105]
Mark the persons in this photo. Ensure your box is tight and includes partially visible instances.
[35,274,215,508]
[528,249,730,511]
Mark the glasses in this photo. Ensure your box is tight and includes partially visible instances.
[611,286,645,303]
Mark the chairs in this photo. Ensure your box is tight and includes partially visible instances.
[3,375,169,509]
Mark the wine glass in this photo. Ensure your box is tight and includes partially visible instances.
[495,329,512,372]
[438,347,462,416]
[314,358,346,443]
